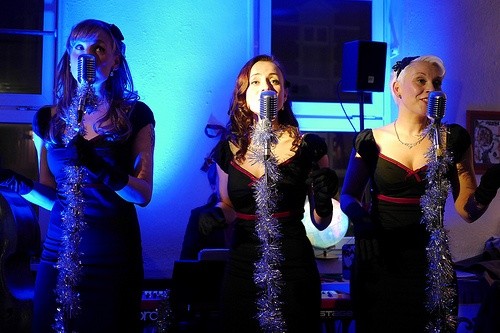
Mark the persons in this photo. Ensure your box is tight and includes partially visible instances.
[0,18,154,333]
[197,55,339,333]
[339,56,500,333]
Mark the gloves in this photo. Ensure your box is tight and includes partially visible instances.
[345,202,375,239]
[67,135,129,191]
[198,206,228,236]
[474,163,500,204]
[309,167,339,217]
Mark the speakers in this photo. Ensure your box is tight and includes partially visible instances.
[340,39,388,93]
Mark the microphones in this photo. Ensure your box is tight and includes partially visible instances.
[78,54,96,84]
[259,90,278,122]
[426,90,447,119]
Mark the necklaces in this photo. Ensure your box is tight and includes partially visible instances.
[393,120,428,149]
